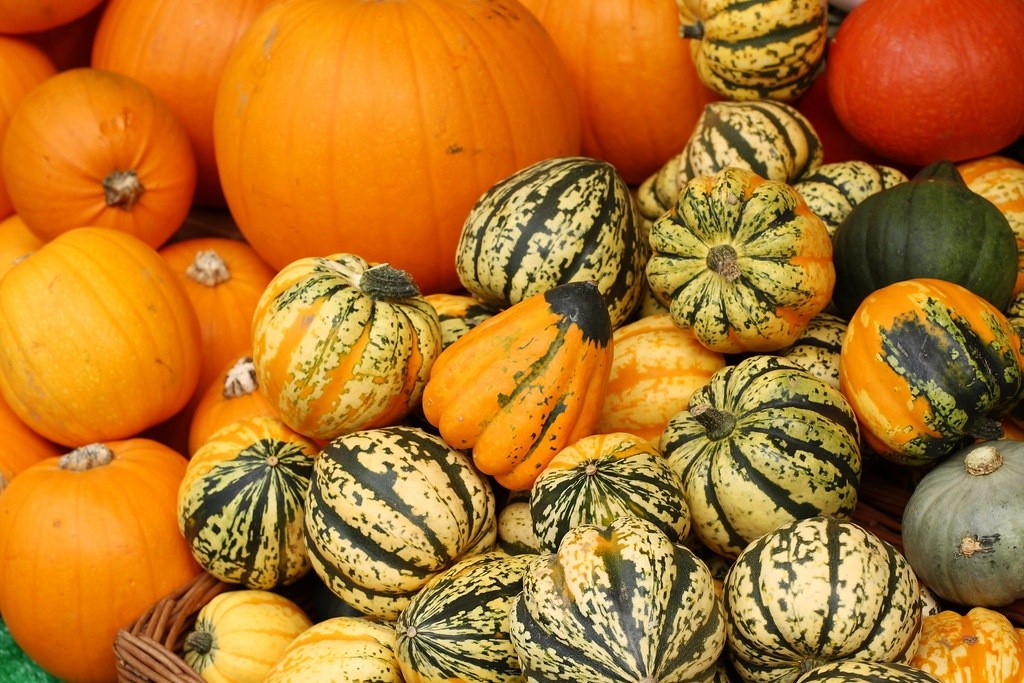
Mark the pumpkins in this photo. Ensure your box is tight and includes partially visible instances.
[0,1,1024,683]
